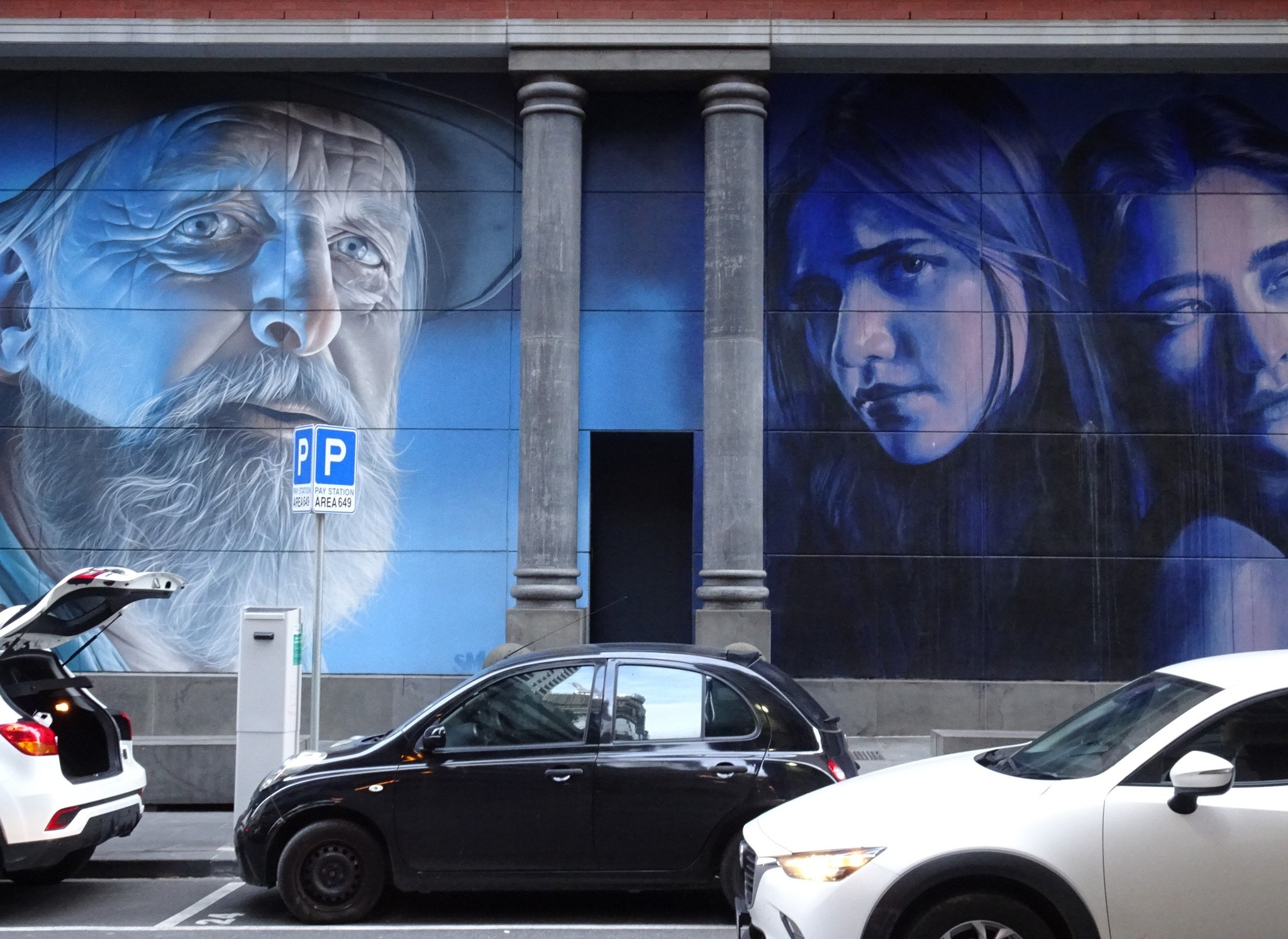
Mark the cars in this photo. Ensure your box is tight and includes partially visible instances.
[735,645,1287,938]
[234,592,860,926]
[0,565,189,883]
